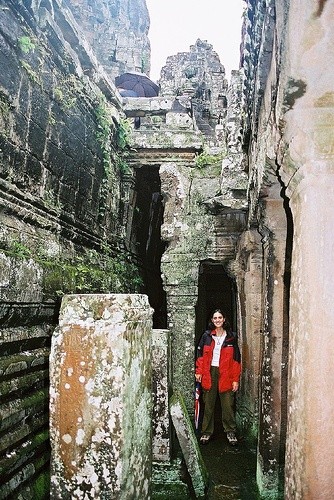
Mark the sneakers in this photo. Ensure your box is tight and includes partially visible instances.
[200,434,212,445]
[227,431,238,446]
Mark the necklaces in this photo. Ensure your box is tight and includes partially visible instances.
[216,330,224,345]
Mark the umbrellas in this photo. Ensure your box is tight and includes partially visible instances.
[194,380,200,430]
[115,72,159,98]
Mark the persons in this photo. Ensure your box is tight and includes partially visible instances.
[195,309,241,444]
